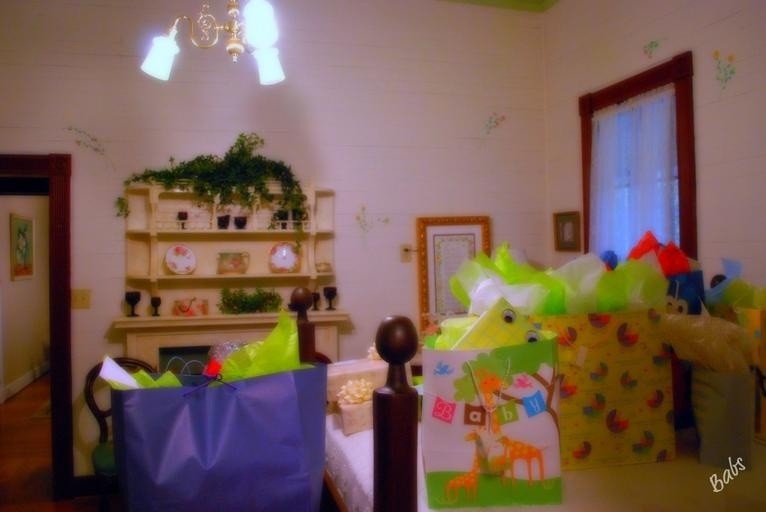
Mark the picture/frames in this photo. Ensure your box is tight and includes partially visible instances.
[553,212,581,252]
[10,213,36,281]
[418,217,489,334]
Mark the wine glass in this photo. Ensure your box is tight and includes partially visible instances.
[178,211,187,231]
[125,289,161,318]
[312,286,338,311]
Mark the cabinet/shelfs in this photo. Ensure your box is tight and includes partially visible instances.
[116,185,348,369]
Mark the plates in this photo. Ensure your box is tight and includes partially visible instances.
[269,241,302,272]
[165,246,196,274]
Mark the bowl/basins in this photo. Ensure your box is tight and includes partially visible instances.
[174,299,208,317]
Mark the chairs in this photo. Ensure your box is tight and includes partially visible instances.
[87,358,162,444]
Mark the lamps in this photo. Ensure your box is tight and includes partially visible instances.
[141,1,288,87]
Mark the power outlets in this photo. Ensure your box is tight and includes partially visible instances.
[71,289,90,309]
[400,244,412,264]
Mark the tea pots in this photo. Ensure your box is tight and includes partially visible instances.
[217,251,251,274]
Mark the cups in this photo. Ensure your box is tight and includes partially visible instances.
[272,209,308,229]
[216,215,249,230]
